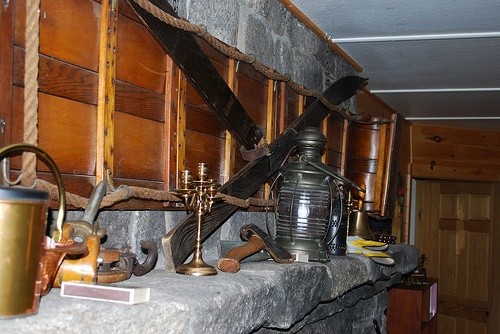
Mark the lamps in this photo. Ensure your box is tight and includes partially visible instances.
[265,125,365,263]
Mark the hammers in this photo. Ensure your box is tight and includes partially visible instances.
[217,222,296,273]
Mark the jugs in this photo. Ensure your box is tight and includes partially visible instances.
[0,143,74,319]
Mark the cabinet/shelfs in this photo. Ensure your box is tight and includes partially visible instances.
[387,278,438,334]
